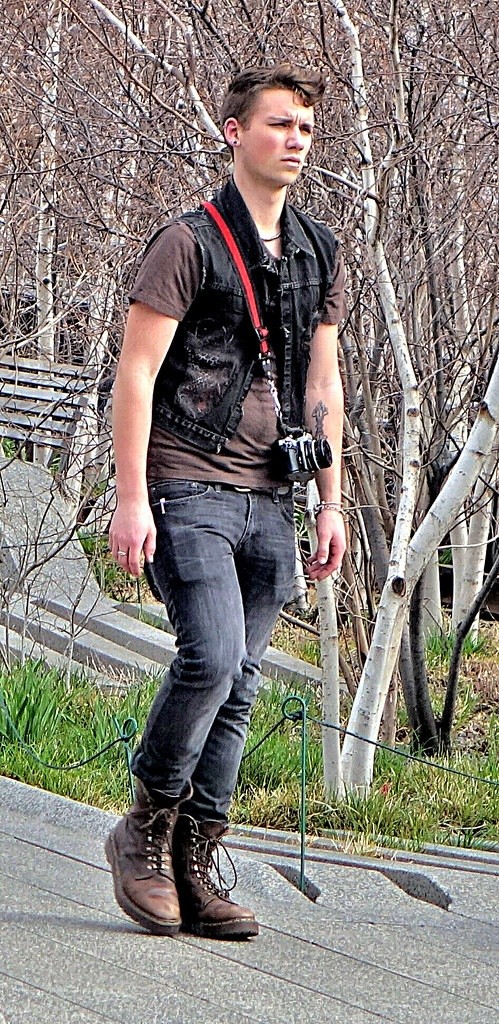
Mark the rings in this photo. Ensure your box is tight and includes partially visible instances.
[117,551,127,556]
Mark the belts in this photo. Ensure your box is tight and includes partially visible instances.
[204,479,291,496]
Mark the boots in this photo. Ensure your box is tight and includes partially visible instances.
[104,782,193,935]
[175,810,260,939]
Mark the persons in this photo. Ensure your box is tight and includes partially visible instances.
[105,63,344,937]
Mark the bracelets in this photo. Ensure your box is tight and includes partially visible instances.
[313,501,345,519]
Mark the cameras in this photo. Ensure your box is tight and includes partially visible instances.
[270,431,332,474]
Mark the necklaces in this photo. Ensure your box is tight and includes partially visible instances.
[261,233,280,242]
